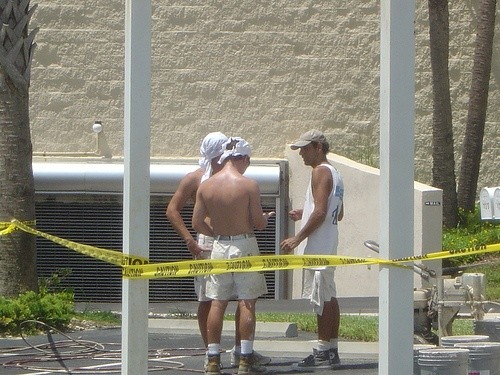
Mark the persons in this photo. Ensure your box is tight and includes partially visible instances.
[165,131,272,371]
[279,129,344,371]
[191,136,276,375]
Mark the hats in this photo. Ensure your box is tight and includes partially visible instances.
[290,129,330,150]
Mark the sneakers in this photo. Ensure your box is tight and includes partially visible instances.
[229,347,272,366]
[237,352,268,374]
[293,348,341,372]
[202,350,223,373]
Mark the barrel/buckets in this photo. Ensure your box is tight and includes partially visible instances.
[454,342,500,375]
[441,335,490,350]
[418,347,470,375]
[413,344,436,375]
